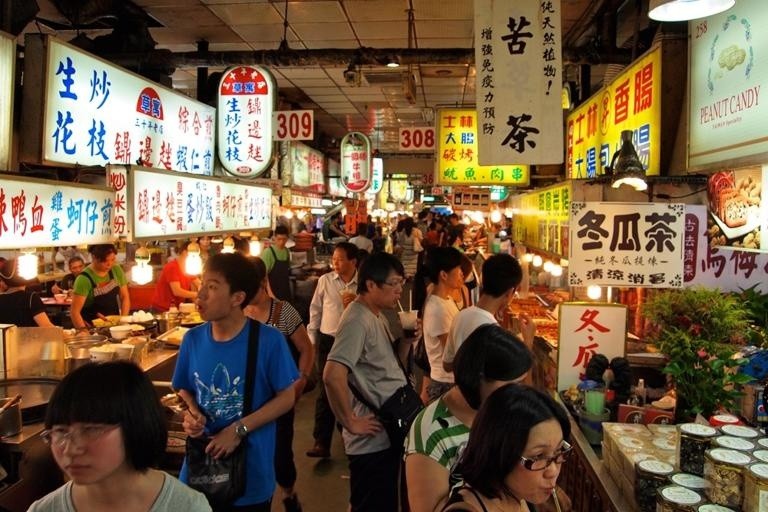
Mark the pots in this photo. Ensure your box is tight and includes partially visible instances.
[0,397,24,437]
[24,302,202,368]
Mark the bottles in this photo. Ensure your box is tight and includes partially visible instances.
[635,378,647,403]
[48,264,53,275]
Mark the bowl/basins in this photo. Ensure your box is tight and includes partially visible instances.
[55,294,67,302]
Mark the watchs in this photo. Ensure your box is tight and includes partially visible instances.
[235,421,247,438]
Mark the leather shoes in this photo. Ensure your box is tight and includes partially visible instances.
[306,440,330,457]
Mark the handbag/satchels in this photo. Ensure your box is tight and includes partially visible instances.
[376,383,425,459]
[185,435,247,510]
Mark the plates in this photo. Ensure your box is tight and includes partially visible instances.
[292,233,313,251]
[708,205,767,239]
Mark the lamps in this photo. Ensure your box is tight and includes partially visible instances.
[16,249,37,280]
[185,236,202,276]
[220,233,235,253]
[131,238,153,285]
[250,231,261,256]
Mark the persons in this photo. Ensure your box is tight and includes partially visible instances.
[306,209,513,403]
[24,360,215,512]
[244,255,314,511]
[305,243,362,458]
[53,244,131,329]
[322,251,422,512]
[169,253,300,512]
[443,254,523,372]
[152,225,292,311]
[1,258,55,327]
[401,323,536,512]
[438,382,575,512]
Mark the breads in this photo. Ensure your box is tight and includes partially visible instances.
[710,167,750,227]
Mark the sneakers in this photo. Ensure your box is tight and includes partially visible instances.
[284,492,302,512]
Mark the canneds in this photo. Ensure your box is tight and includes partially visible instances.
[635,414,768,512]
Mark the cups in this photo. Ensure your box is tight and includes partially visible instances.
[491,244,500,253]
[398,310,420,344]
[578,404,610,445]
[337,288,354,309]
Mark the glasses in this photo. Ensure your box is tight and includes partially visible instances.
[386,278,405,287]
[517,440,573,471]
[40,423,122,449]
